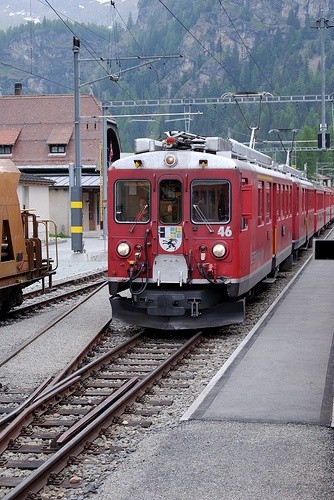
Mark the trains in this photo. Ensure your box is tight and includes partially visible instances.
[0,151,59,314]
[100,128,334,332]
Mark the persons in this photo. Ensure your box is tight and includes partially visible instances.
[128,197,149,223]
